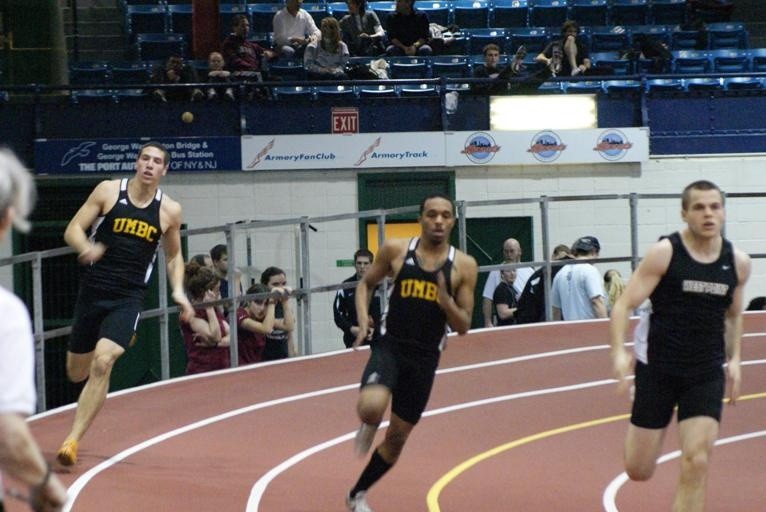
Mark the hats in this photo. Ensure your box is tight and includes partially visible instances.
[573,237,599,251]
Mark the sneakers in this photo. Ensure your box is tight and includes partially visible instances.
[511,44,526,74]
[58,440,78,466]
[552,46,563,73]
[345,490,370,512]
[356,424,378,452]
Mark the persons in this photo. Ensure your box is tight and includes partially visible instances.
[2,145,72,512]
[746,295,766,310]
[608,178,751,512]
[55,138,192,468]
[474,44,563,94]
[148,15,274,102]
[274,0,431,79]
[479,235,624,330]
[534,20,597,78]
[345,190,481,512]
[332,248,381,349]
[170,245,305,378]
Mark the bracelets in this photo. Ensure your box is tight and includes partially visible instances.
[33,457,55,496]
